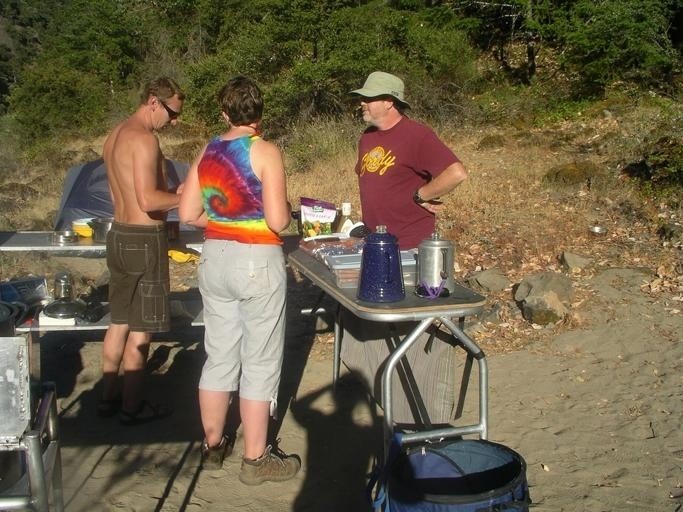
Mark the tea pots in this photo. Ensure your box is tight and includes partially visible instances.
[414,232,456,299]
[355,223,406,303]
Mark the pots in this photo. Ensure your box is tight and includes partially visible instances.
[86,216,115,242]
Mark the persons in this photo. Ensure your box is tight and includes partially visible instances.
[177,74,301,486]
[345,70,466,254]
[95,76,183,425]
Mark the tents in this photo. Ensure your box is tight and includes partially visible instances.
[52,158,189,232]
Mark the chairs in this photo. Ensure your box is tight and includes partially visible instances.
[0,332,66,512]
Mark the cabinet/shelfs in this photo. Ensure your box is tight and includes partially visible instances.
[1,223,207,398]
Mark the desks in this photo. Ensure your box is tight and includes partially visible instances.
[279,217,491,474]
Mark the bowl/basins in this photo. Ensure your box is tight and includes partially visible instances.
[72,218,96,238]
[166,220,180,241]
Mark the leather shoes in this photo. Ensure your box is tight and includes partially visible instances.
[239,444,301,485]
[201,431,234,469]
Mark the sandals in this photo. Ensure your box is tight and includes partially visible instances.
[119,398,173,424]
[97,394,122,417]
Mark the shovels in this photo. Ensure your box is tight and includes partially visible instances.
[307,225,371,243]
[303,219,354,242]
[318,222,364,240]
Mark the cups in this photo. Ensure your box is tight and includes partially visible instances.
[63,231,71,238]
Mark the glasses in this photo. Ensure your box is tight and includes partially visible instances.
[160,101,180,118]
[415,280,451,299]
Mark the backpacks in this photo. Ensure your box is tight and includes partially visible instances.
[366,438,531,511]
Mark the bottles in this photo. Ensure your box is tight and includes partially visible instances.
[53,272,71,300]
[335,202,354,233]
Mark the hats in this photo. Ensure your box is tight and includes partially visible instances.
[348,71,412,110]
